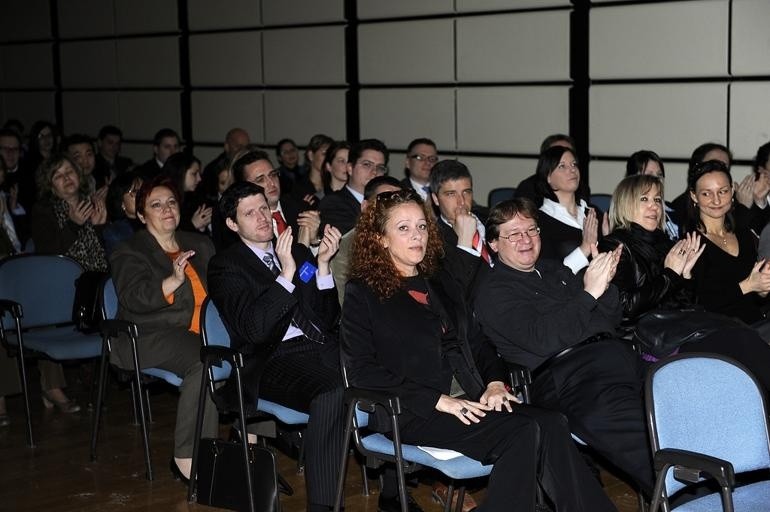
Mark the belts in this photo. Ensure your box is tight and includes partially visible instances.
[529,333,609,380]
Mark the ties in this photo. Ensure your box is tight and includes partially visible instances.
[472,230,495,269]
[271,210,288,235]
[263,251,325,345]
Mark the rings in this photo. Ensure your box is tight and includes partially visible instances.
[677,250,686,256]
[691,249,697,254]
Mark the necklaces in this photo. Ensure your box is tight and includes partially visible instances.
[696,229,730,250]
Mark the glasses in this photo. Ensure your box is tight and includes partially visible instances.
[354,160,386,174]
[498,227,541,241]
[411,152,437,162]
[376,188,416,218]
[246,171,280,185]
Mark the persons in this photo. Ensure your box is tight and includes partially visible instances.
[598,174,770,441]
[0,114,490,511]
[752,141,770,210]
[476,196,661,511]
[626,148,671,206]
[673,143,770,247]
[511,134,591,204]
[535,145,606,276]
[336,191,621,511]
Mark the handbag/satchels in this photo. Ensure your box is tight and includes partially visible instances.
[634,306,754,359]
[195,437,293,511]
[71,270,109,335]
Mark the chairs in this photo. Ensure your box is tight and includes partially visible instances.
[1,252,105,449]
[68,251,641,510]
[642,351,770,512]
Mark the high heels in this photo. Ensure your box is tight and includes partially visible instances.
[169,457,197,486]
[227,427,259,447]
[41,392,80,413]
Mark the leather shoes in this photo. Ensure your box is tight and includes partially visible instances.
[378,491,423,512]
[0,412,9,426]
